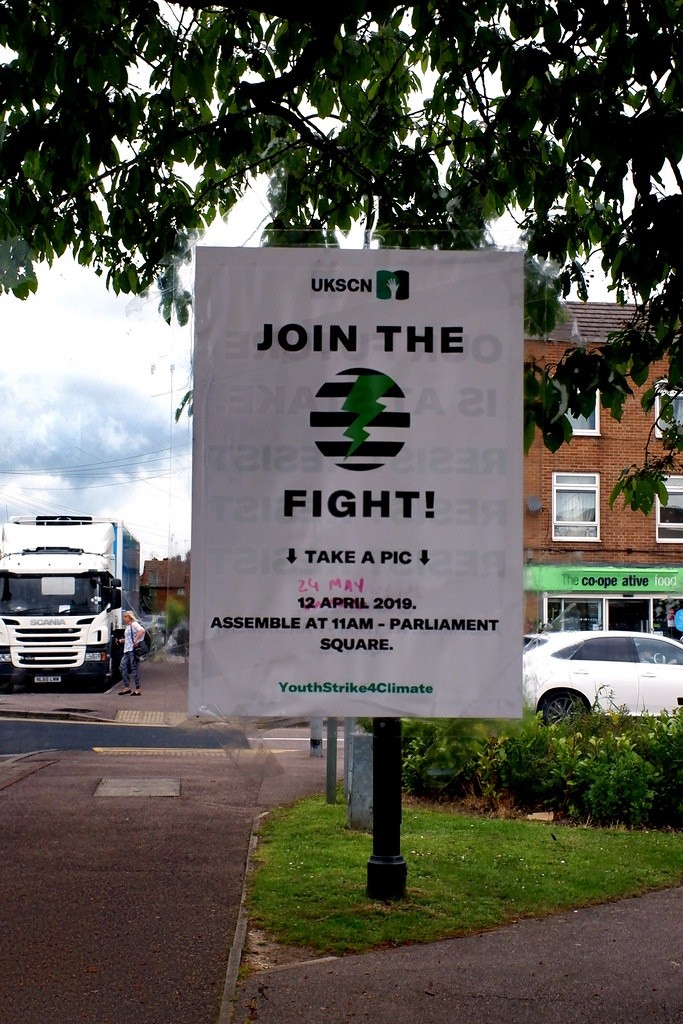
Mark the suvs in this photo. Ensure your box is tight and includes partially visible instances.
[523,629,683,725]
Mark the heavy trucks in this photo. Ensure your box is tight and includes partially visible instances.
[0,515,140,692]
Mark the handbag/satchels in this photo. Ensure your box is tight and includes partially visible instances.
[133,641,149,657]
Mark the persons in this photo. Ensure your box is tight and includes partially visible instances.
[118,611,145,696]
[637,644,677,664]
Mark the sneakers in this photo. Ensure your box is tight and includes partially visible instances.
[130,692,141,696]
[118,690,131,695]
